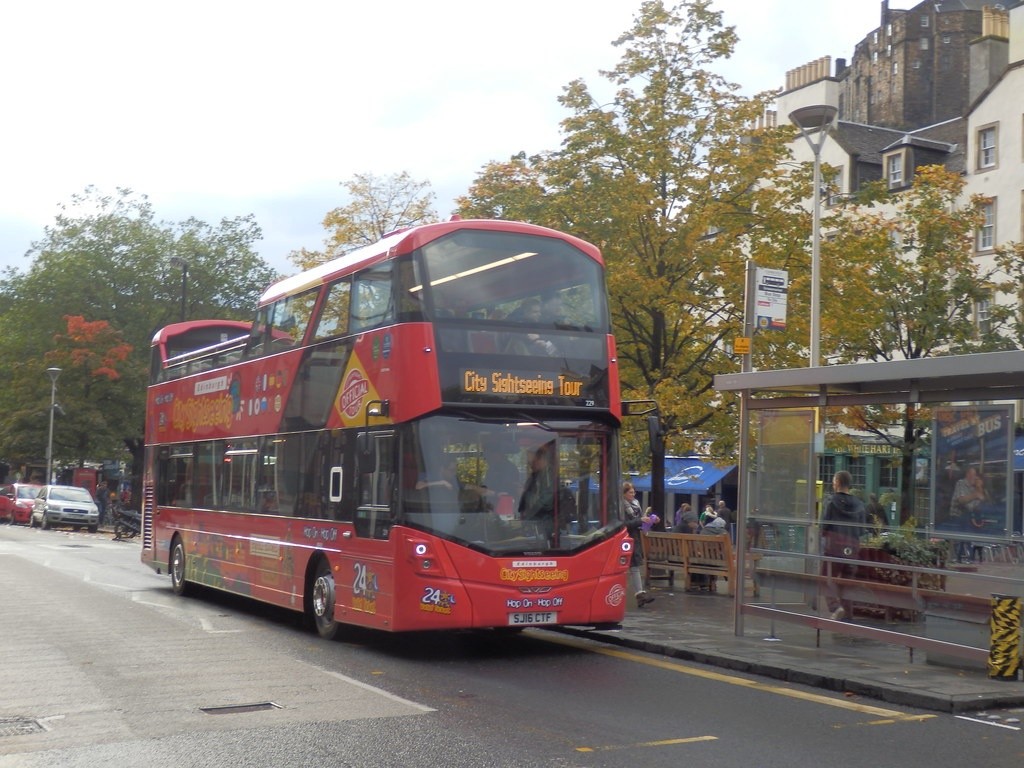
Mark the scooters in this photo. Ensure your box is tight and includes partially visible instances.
[113,508,143,540]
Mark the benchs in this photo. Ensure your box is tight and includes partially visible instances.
[168,337,295,381]
[640,530,762,597]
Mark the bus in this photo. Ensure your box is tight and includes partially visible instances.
[135,214,671,647]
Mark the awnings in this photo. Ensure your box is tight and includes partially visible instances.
[565,458,739,495]
[1013,436,1024,473]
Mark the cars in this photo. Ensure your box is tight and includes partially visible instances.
[0,483,43,525]
[28,484,100,534]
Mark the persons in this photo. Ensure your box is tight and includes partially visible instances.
[642,506,660,531]
[951,540,983,564]
[822,470,866,624]
[622,482,660,607]
[673,501,731,592]
[865,493,889,534]
[415,455,508,497]
[518,444,560,520]
[95,480,110,524]
[745,517,766,598]
[484,448,519,494]
[949,467,985,518]
[499,290,567,357]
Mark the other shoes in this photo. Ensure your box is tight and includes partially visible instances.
[831,607,844,620]
[636,593,654,607]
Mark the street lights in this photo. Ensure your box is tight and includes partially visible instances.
[789,105,838,367]
[42,367,65,486]
[167,254,189,322]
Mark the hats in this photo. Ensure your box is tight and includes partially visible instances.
[712,517,726,527]
[683,512,697,522]
